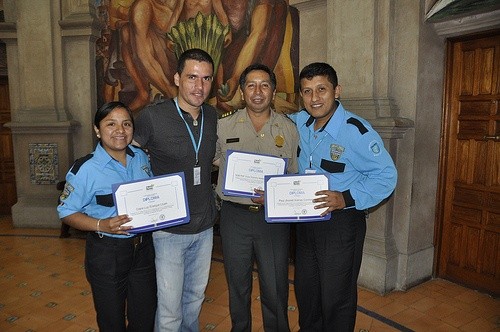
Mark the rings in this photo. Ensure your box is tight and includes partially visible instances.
[118,225,121,230]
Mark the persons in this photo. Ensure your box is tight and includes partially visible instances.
[57,102,155,332]
[214,63,300,332]
[285,62,398,332]
[131,48,223,332]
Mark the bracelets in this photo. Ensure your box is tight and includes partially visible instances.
[93,219,108,239]
